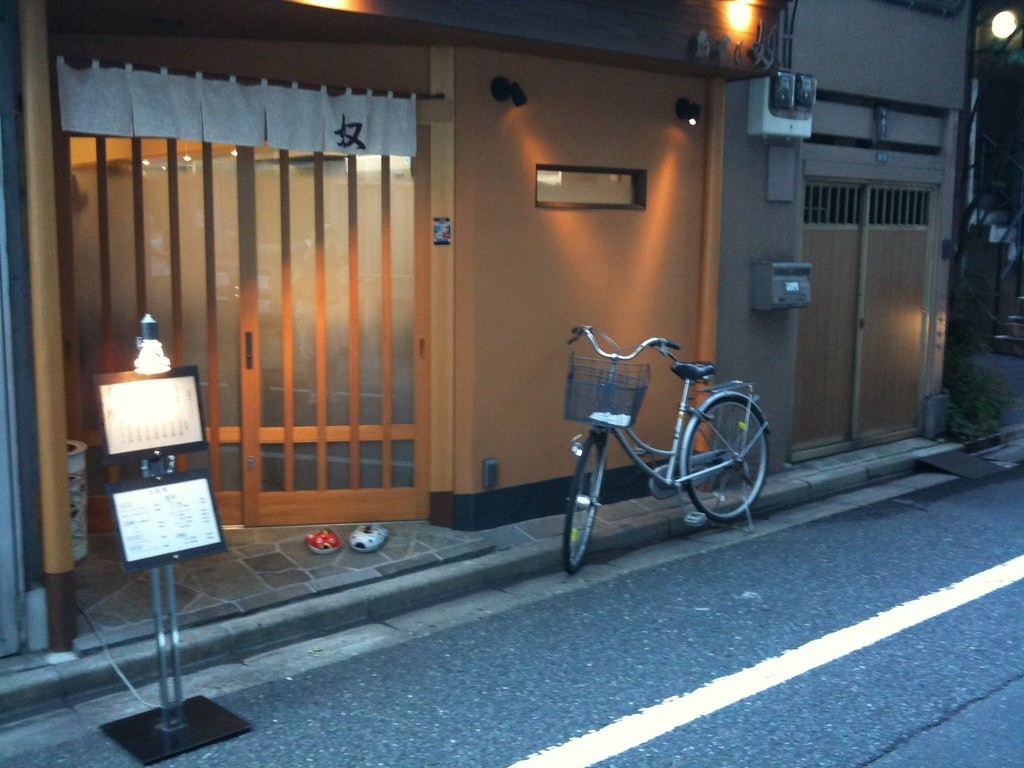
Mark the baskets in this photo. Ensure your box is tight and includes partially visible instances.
[563,351,651,428]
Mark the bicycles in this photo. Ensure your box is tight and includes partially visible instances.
[561,326,773,574]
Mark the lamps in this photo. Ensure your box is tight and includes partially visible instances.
[491,75,527,108]
[676,98,700,126]
